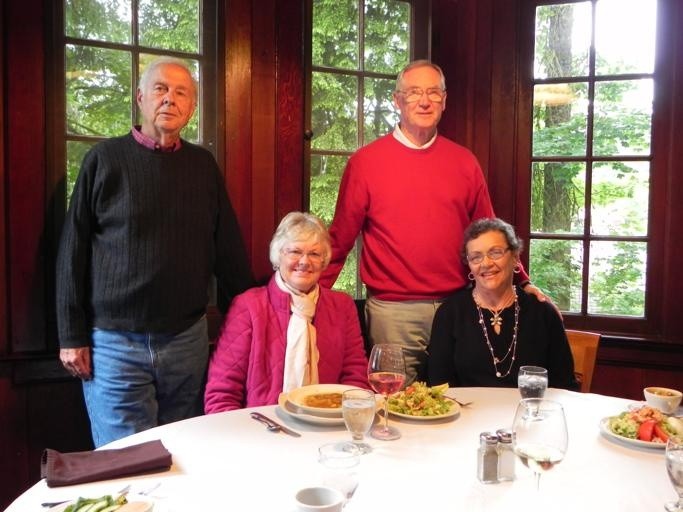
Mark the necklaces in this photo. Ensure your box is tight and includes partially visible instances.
[471,279,522,379]
[478,290,514,337]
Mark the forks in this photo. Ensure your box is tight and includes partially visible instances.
[40,485,131,506]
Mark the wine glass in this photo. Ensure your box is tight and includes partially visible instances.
[317,443,360,511]
[340,389,376,453]
[664,438,683,512]
[367,343,406,440]
[512,398,568,490]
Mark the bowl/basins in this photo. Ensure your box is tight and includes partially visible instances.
[643,387,682,414]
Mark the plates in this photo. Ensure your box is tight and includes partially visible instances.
[49,495,152,511]
[276,383,383,426]
[382,392,459,419]
[599,415,682,448]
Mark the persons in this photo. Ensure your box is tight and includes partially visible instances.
[204,210,374,414]
[427,214,581,393]
[317,60,566,391]
[56,57,254,448]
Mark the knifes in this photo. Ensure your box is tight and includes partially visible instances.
[251,411,300,438]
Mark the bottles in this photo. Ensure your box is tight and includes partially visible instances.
[478,428,515,481]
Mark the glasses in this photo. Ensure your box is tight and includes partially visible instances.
[397,88,448,103]
[283,244,326,266]
[466,245,511,264]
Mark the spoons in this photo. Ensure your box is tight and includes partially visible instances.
[251,414,279,430]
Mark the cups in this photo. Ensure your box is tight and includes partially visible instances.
[295,487,342,511]
[517,366,547,421]
